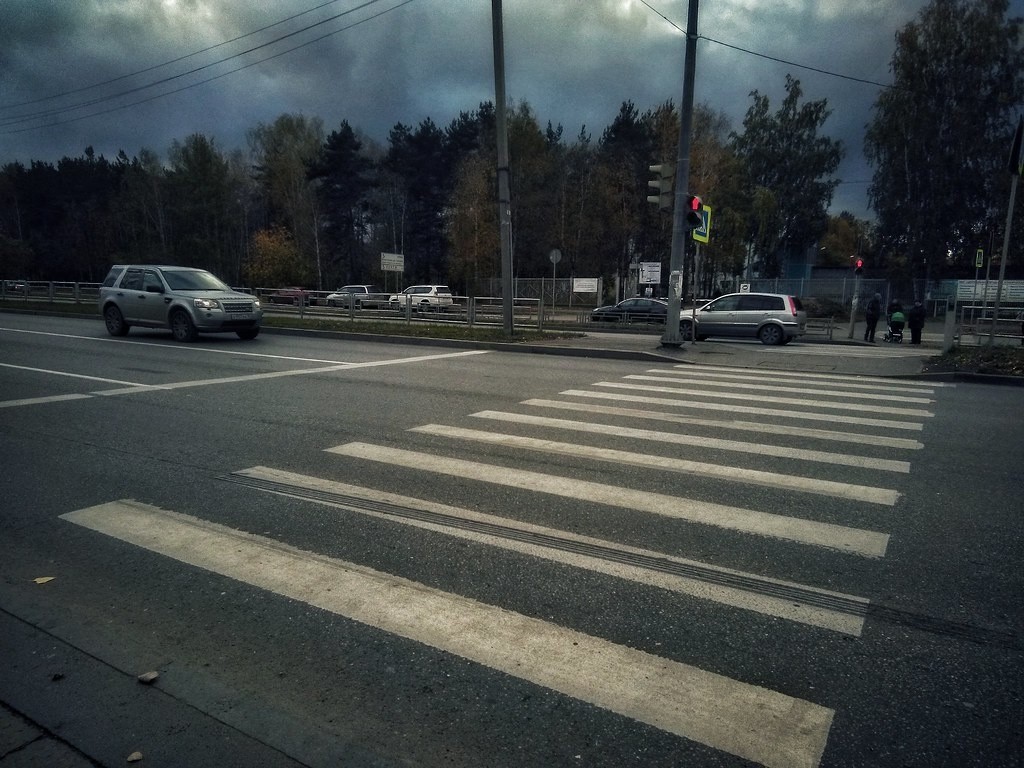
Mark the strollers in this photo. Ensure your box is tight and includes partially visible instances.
[883,312,905,344]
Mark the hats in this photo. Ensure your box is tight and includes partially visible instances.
[913,298,922,303]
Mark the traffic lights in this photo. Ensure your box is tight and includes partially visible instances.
[686,195,703,228]
[647,164,676,213]
[854,259,864,276]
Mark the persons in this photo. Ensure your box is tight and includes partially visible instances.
[864,293,881,343]
[908,299,925,345]
[887,297,905,330]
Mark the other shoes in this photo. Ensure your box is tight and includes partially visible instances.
[865,338,868,341]
[869,340,876,343]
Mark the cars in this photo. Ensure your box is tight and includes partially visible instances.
[679,293,807,345]
[388,285,453,312]
[325,285,385,310]
[268,287,317,306]
[590,298,668,325]
[97,265,263,343]
